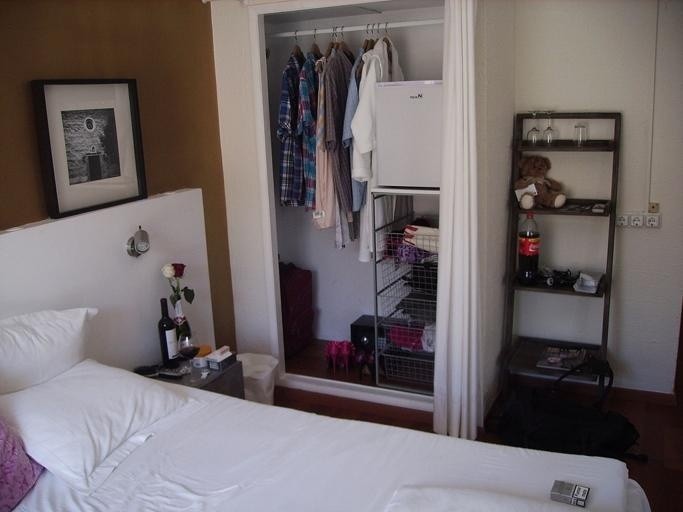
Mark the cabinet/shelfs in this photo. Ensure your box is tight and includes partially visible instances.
[499,111,623,408]
[369,186,440,396]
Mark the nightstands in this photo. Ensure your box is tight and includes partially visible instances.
[135,352,246,400]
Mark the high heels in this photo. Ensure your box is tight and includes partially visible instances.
[324,340,352,378]
[357,352,377,383]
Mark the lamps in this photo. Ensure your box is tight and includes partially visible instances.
[127,225,150,258]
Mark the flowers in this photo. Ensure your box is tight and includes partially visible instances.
[161,262,195,309]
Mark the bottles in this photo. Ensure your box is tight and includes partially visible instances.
[518,210,540,286]
[158,297,180,369]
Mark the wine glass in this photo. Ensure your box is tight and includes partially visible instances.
[177,331,200,375]
[527,110,554,148]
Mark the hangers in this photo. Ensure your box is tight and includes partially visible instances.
[291,22,389,43]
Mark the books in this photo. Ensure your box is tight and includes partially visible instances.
[535,345,588,373]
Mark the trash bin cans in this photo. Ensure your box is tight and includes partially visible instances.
[236,353,279,405]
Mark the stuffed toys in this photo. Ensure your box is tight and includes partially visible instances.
[510,154,568,211]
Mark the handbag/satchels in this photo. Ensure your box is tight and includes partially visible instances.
[279,261,313,358]
[484,358,649,463]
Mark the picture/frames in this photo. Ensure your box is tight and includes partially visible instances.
[30,77,150,220]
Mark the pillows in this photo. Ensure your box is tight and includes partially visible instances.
[0,416,46,512]
[0,308,100,394]
[0,358,206,496]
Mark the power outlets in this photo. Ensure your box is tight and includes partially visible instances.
[616,214,629,227]
[645,213,662,228]
[630,215,645,227]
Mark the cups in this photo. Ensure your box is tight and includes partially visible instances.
[573,126,587,149]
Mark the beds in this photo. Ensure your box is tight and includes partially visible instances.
[11,380,652,512]
